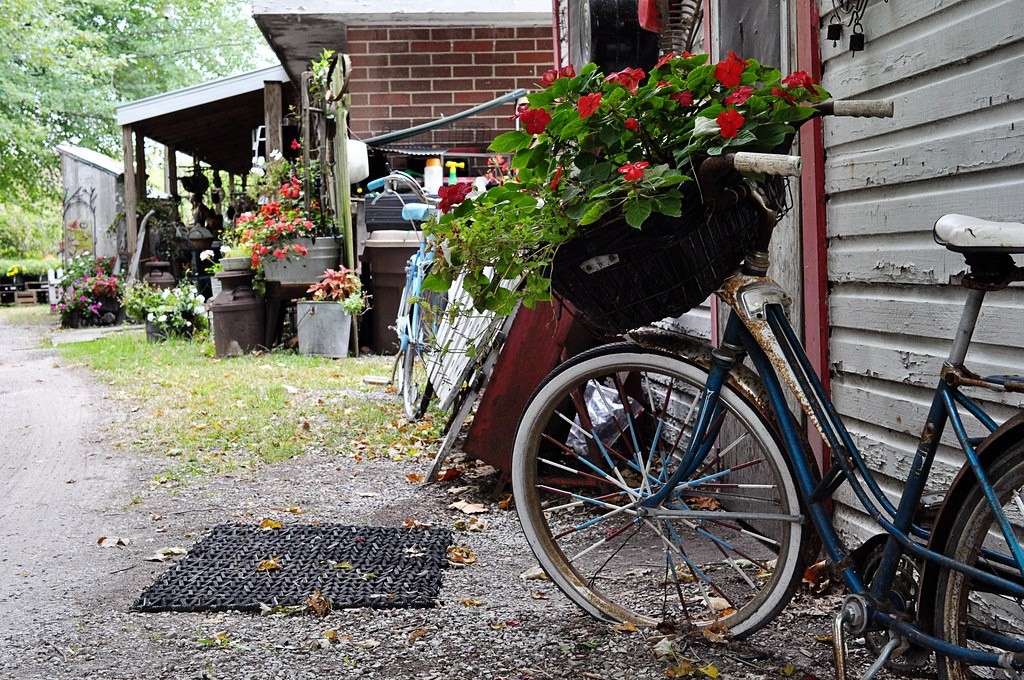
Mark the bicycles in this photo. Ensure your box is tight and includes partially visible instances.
[362,165,472,422]
[512,97,1024,679]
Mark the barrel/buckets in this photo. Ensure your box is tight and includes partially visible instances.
[294,300,352,359]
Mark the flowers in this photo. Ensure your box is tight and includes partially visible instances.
[395,46,832,369]
[1,137,371,329]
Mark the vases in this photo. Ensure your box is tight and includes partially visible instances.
[295,299,353,360]
[142,309,194,344]
